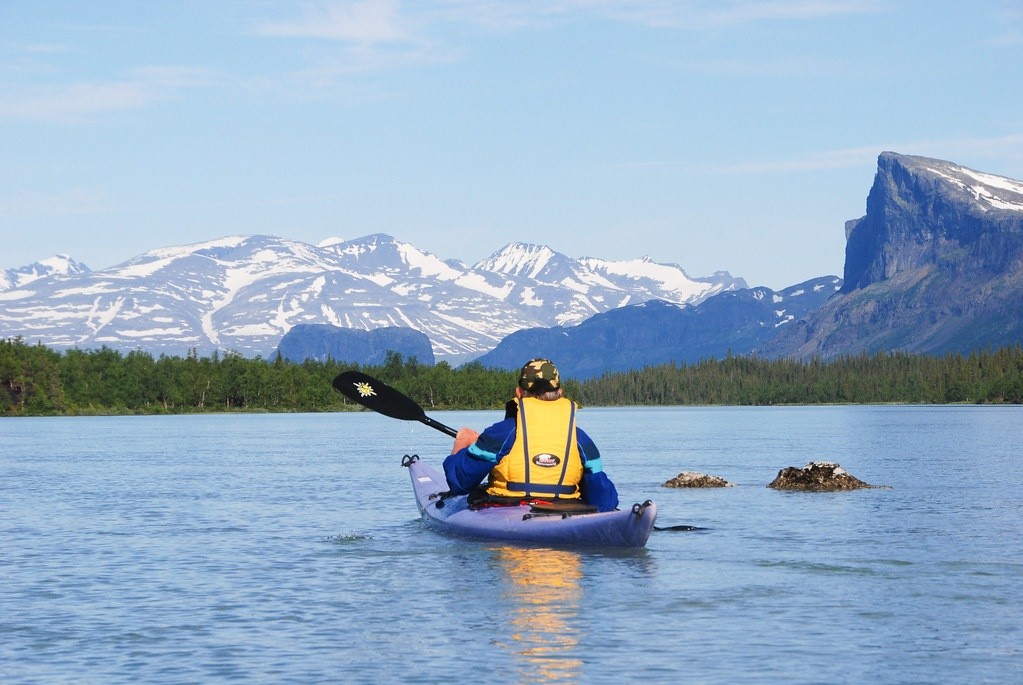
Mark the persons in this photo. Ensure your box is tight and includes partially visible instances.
[442,358,619,510]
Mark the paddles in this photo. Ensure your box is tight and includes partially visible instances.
[329,371,483,446]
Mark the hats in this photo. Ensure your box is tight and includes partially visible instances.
[518,358,560,393]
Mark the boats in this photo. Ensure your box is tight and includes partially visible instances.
[401,454,658,548]
